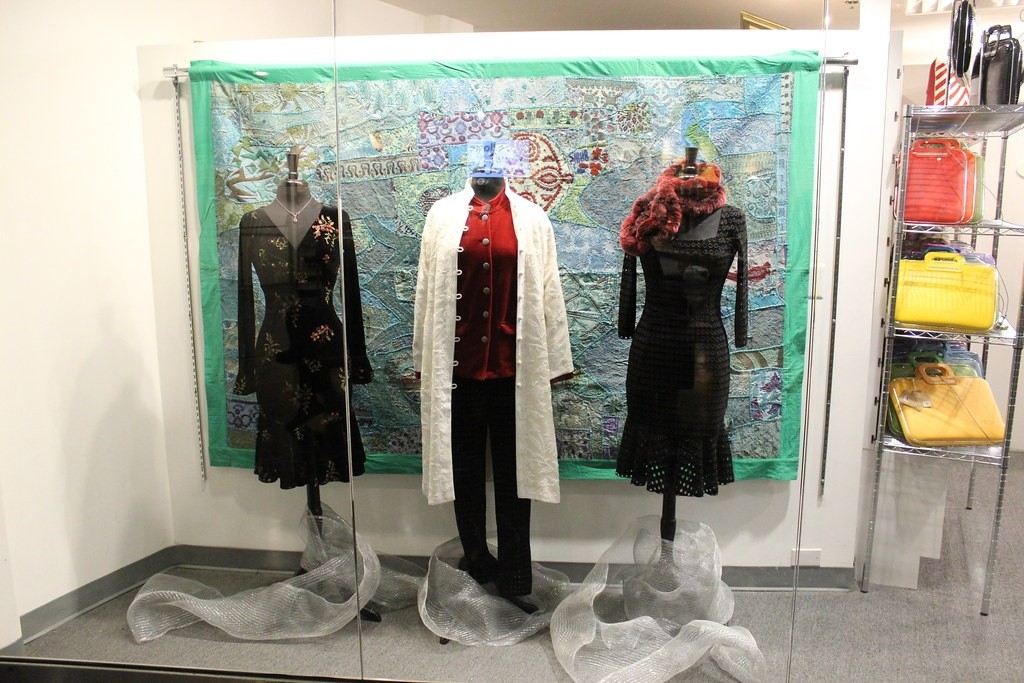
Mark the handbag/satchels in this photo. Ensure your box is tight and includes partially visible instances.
[895,234,998,333]
[887,340,1005,447]
[951,0,973,78]
[896,138,983,224]
[969,24,1024,104]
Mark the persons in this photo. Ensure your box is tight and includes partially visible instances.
[411,168,573,594]
[233,179,375,489]
[616,156,748,497]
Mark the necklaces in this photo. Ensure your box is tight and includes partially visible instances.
[275,195,314,223]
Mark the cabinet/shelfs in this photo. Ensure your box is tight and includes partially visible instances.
[860,103,1024,618]
